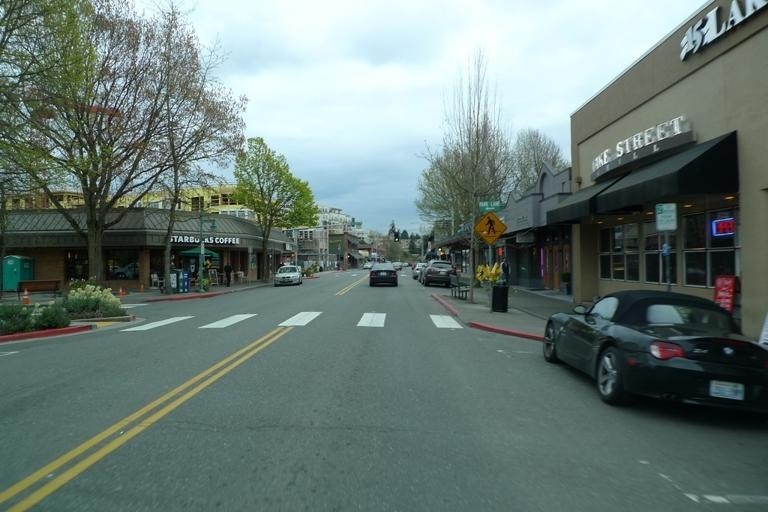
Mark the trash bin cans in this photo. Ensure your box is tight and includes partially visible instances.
[493,279,508,312]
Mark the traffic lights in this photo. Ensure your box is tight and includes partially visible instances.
[394,232,399,242]
[498,246,504,256]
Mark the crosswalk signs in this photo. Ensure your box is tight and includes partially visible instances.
[475,212,507,243]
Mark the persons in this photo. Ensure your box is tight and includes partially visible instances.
[224,262,232,287]
[500,256,512,287]
[278,261,285,268]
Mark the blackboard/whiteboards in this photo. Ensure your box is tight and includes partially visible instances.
[208,269,218,284]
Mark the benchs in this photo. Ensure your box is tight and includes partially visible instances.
[449,274,470,300]
[17,281,60,298]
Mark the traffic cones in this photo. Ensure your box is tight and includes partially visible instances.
[140,282,144,292]
[23,287,30,305]
[119,287,123,295]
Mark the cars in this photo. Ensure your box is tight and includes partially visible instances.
[412,259,456,287]
[363,260,406,287]
[274,265,302,286]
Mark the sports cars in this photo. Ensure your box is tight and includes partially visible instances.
[542,289,768,416]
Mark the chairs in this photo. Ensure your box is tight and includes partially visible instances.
[208,269,249,287]
[151,273,165,287]
